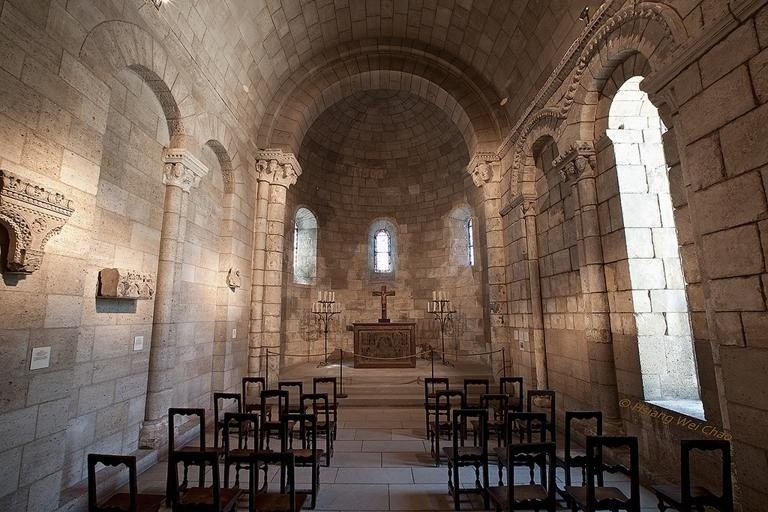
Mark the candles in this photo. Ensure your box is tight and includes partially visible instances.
[425,290,455,312]
[311,289,342,313]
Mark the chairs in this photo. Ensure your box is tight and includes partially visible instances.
[424,376,640,510]
[652,439,735,510]
[87,453,166,512]
[167,377,338,511]
